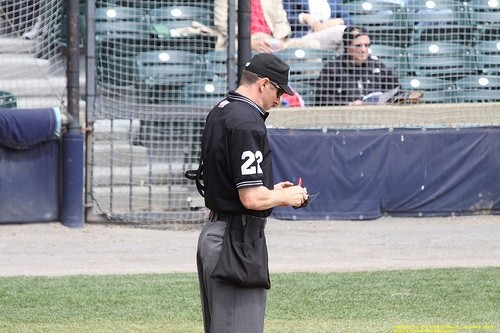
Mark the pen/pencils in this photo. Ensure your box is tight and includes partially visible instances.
[297,177,304,188]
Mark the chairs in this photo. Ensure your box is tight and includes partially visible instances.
[98,0,500,105]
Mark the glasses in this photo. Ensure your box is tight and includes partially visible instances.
[354,44,372,48]
[268,81,285,98]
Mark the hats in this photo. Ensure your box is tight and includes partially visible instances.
[343,27,370,52]
[244,53,294,95]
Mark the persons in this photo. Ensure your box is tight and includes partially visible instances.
[314,26,406,106]
[212,0,348,78]
[185,53,309,333]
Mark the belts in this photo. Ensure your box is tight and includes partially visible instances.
[208,210,267,226]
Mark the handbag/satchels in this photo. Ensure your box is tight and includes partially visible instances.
[210,214,271,290]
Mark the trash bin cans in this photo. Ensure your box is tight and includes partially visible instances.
[0,90,17,110]
